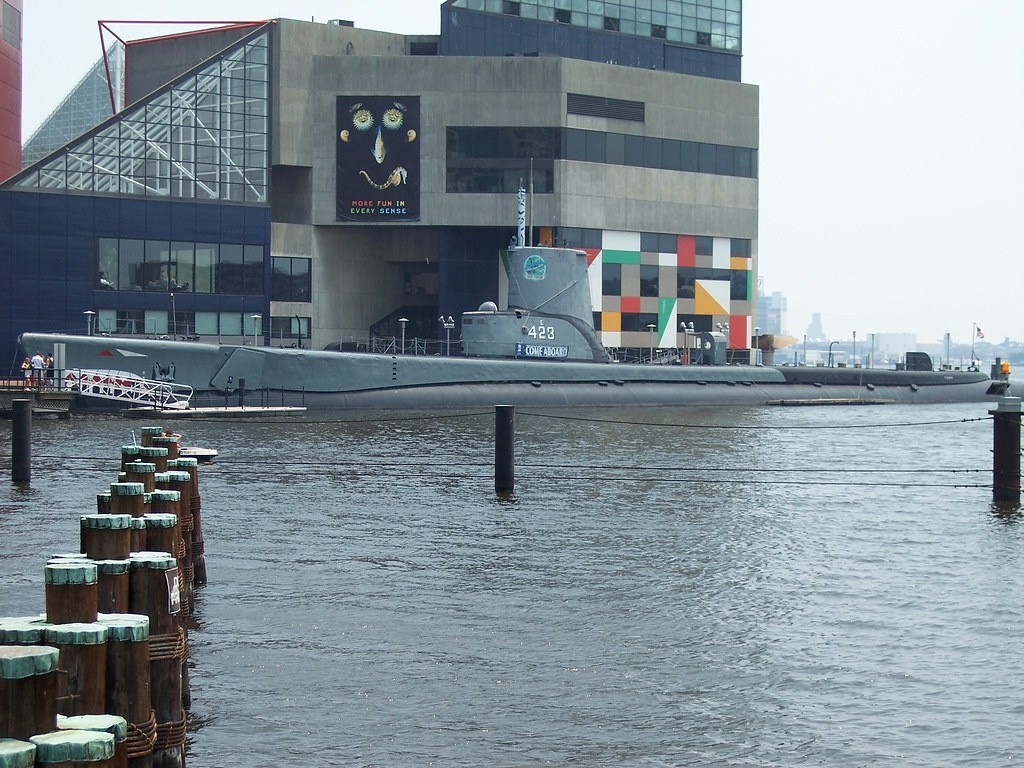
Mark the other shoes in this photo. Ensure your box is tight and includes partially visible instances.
[25,388,54,392]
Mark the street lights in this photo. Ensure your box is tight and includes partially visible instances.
[438,316,455,357]
[251,314,262,346]
[754,326,761,366]
[82,310,96,336]
[828,340,839,367]
[398,318,409,353]
[681,321,695,364]
[647,323,657,362]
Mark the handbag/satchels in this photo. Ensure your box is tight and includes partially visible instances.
[47,358,54,368]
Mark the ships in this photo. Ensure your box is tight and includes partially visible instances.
[16,157,1024,412]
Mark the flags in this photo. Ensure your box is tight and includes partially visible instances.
[976,327,984,338]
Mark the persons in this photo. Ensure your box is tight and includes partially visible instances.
[676,353,682,365]
[100,270,180,292]
[28,351,54,391]
[21,358,31,392]
[162,430,181,450]
